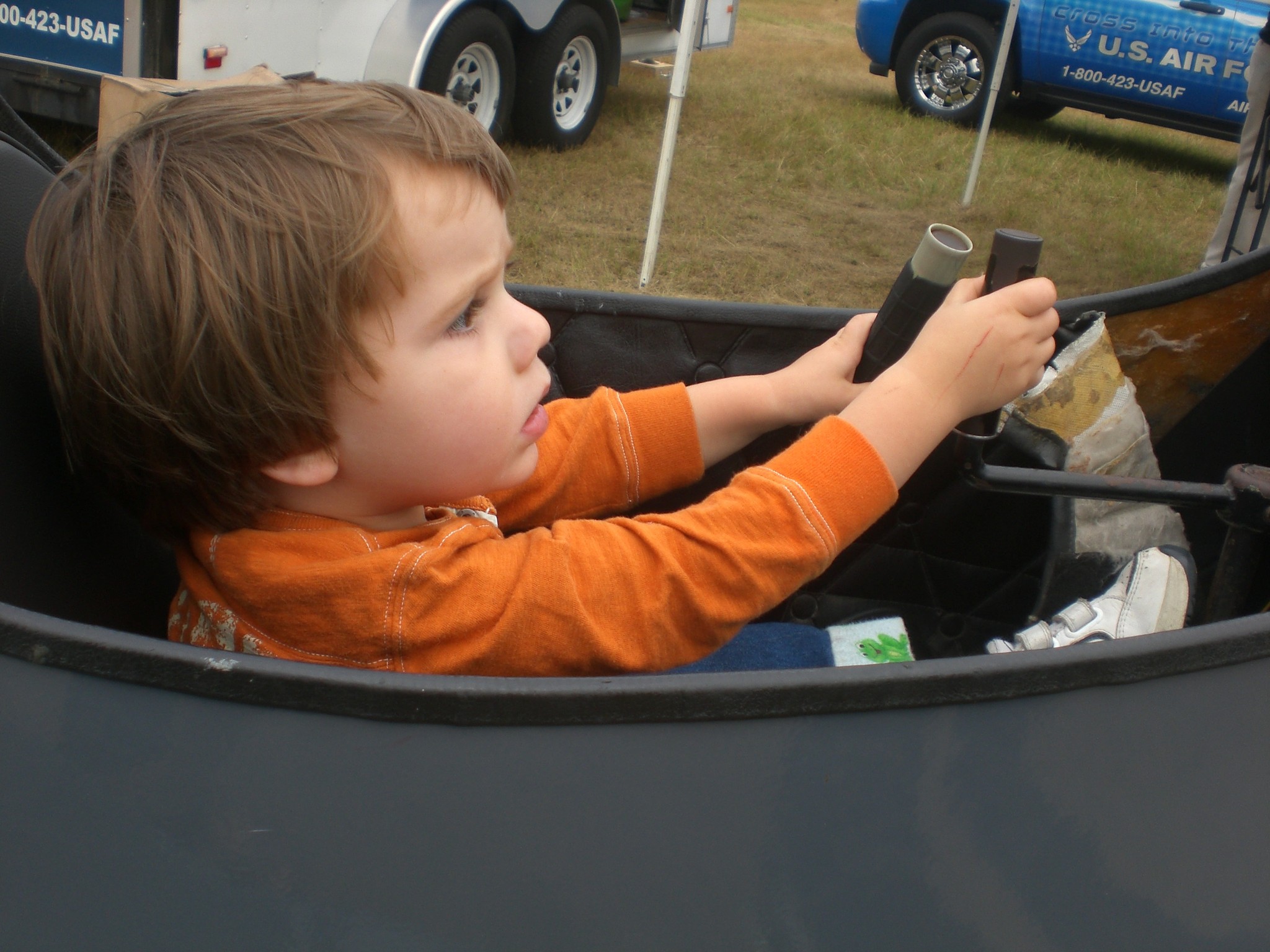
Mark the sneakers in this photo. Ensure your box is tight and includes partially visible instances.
[985,543,1197,654]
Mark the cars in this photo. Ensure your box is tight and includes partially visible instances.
[851,1,1270,153]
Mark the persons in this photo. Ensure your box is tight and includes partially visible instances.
[30,79,1191,676]
[1198,10,1270,272]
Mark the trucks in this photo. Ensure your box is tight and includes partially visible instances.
[1,1,744,157]
